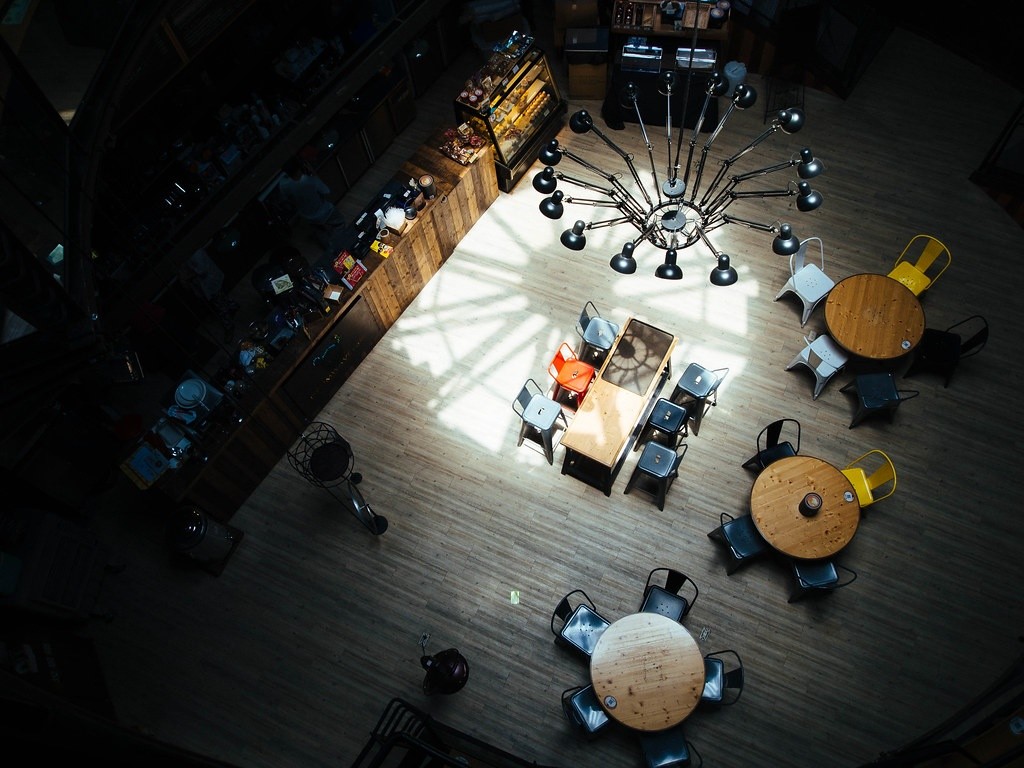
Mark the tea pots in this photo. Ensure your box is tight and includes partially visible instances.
[403,198,417,220]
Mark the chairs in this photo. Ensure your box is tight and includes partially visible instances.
[786,336,850,402]
[546,343,596,412]
[623,442,687,511]
[511,378,568,465]
[561,685,610,736]
[840,370,921,430]
[741,419,803,473]
[786,559,859,600]
[886,234,953,298]
[635,397,702,449]
[838,448,897,508]
[669,360,729,437]
[773,238,835,329]
[638,729,705,766]
[575,301,620,366]
[641,568,700,625]
[700,649,747,706]
[549,588,612,661]
[904,313,991,389]
[708,512,766,575]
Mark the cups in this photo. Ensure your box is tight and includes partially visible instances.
[461,88,484,109]
[379,228,391,244]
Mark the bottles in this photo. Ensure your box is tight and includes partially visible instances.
[614,0,644,27]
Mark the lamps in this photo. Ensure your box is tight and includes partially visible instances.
[531,2,827,286]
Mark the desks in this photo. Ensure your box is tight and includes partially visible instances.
[558,316,681,499]
[825,272,926,359]
[749,456,861,555]
[91,280,382,521]
[589,611,706,731]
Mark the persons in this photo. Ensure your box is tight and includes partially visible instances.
[279,154,347,244]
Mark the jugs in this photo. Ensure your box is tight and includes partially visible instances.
[418,173,437,200]
[798,492,823,517]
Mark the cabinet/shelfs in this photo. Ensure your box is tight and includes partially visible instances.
[602,25,722,134]
[454,27,569,193]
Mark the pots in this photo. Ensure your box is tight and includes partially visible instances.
[162,504,234,567]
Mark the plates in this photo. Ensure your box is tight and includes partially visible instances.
[174,378,207,410]
